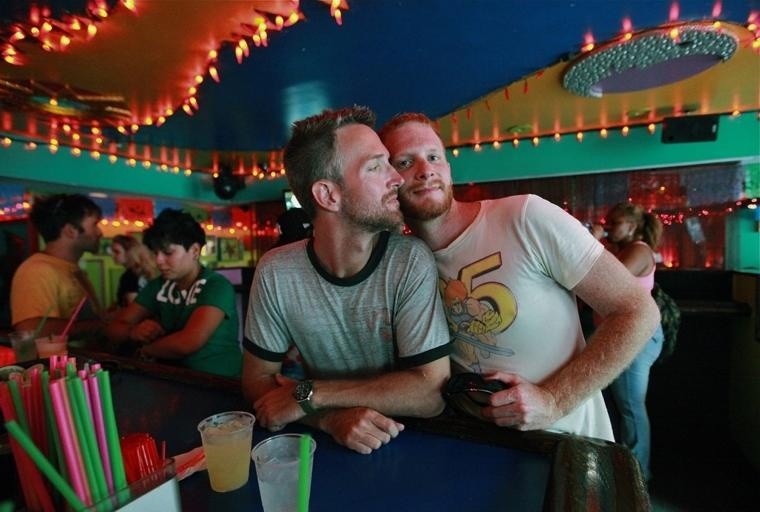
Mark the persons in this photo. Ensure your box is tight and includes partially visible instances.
[112,235,154,307]
[106,208,245,380]
[241,105,453,456]
[377,112,663,443]
[590,202,668,482]
[0,232,26,332]
[11,193,117,343]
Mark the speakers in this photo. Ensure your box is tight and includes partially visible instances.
[662,114,718,143]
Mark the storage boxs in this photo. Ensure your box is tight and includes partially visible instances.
[725,206,760,274]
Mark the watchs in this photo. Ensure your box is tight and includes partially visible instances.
[293,379,320,417]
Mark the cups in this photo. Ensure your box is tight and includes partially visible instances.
[251,433,316,512]
[196,411,256,492]
[7,329,41,363]
[36,335,70,360]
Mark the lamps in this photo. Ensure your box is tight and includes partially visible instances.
[562,26,736,100]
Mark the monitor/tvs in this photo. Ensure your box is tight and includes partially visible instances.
[283,188,303,212]
